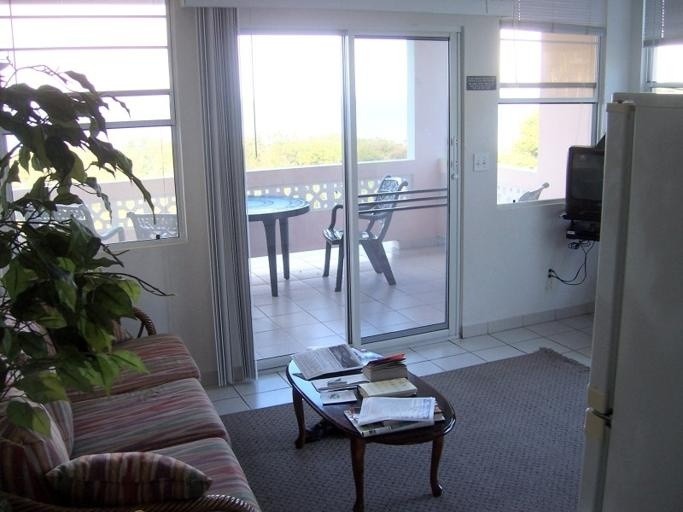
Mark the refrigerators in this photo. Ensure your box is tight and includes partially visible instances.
[575,90,683,512]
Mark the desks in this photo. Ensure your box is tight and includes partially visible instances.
[247,195,310,297]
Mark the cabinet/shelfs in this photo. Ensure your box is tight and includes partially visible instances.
[323,175,407,292]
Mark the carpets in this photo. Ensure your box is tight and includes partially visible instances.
[220,346,589,511]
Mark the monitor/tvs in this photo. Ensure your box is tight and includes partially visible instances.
[565,146,604,218]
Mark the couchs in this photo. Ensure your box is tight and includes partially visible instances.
[1,304,261,511]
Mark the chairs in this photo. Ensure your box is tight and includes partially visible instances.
[23,204,125,243]
[128,213,179,240]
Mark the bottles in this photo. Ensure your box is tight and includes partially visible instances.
[519,182,549,203]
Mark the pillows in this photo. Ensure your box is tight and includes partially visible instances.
[45,451,213,501]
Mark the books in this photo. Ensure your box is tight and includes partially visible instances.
[292,343,445,439]
